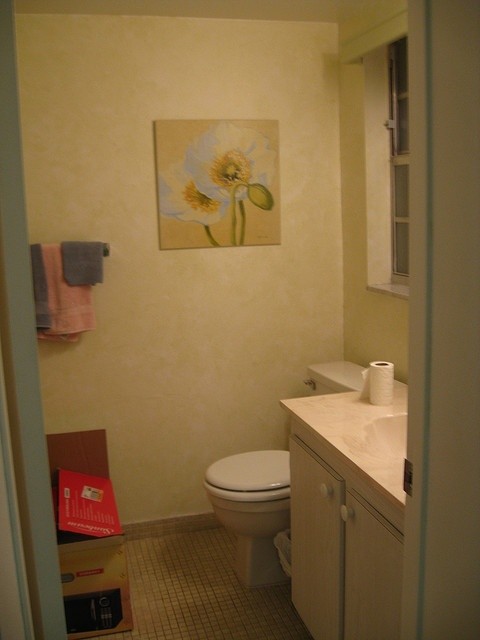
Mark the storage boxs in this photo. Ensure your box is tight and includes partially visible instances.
[45,426,136,635]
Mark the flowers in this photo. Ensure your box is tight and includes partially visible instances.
[158,123,279,248]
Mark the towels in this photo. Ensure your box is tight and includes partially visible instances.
[61,240,103,286]
[31,243,96,342]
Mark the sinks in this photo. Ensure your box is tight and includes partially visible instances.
[365,414,406,469]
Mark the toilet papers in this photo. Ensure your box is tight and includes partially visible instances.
[360,361,394,406]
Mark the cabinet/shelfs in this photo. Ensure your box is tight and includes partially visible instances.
[290,421,345,640]
[344,487,404,640]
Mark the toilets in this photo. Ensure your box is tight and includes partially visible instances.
[205,359,369,588]
